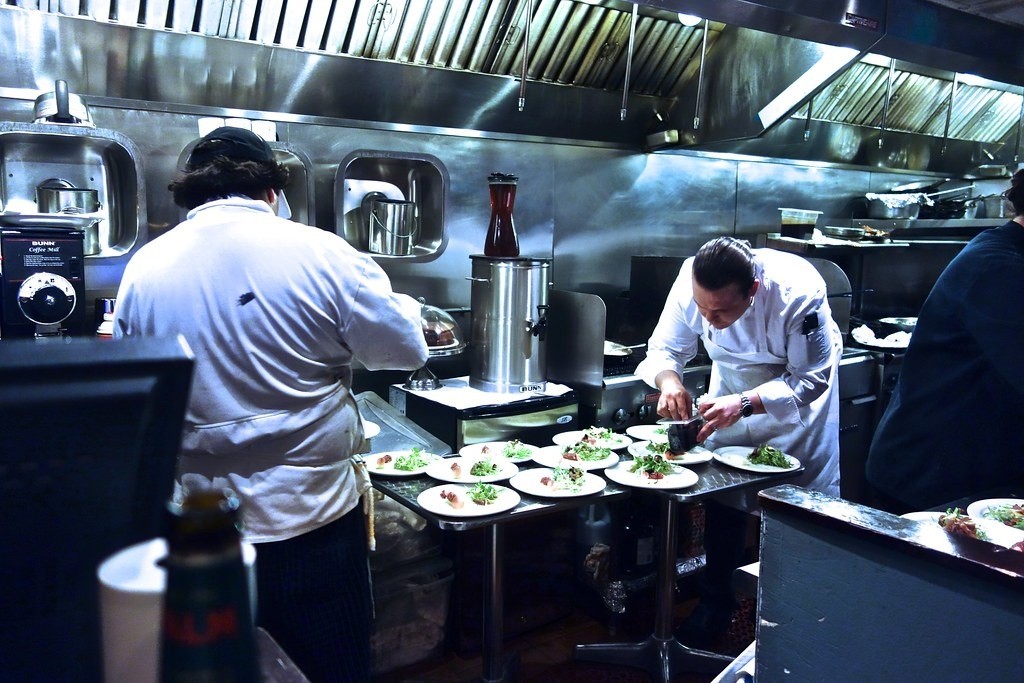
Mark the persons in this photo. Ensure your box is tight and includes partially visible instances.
[863,169,1024,518]
[634,236,843,648]
[113,127,429,683]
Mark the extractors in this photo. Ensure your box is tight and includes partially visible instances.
[594,5,1024,176]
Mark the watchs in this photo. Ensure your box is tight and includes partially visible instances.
[739,391,753,418]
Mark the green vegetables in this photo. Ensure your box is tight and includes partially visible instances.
[748,442,794,469]
[942,505,1024,542]
[393,427,675,505]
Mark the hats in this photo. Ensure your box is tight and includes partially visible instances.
[186,127,292,219]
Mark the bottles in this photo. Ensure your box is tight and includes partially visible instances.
[154,485,263,683]
[483,171,520,256]
[96,313,113,339]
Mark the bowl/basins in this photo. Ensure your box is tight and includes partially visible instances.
[877,317,918,339]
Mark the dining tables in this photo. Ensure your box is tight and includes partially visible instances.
[573,439,805,683]
[371,461,633,683]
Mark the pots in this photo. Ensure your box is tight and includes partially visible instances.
[860,178,1012,219]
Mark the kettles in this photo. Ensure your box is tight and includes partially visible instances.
[33,79,92,125]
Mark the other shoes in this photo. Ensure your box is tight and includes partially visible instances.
[679,602,732,647]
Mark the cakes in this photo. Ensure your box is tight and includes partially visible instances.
[423,323,456,347]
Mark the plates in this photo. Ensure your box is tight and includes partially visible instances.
[531,444,620,471]
[604,461,699,489]
[628,441,713,464]
[967,498,1024,531]
[713,446,801,473]
[417,484,521,517]
[900,512,1022,553]
[552,432,631,450]
[510,468,606,498]
[364,451,441,476]
[425,456,519,484]
[626,424,671,441]
[460,442,538,463]
[362,420,381,440]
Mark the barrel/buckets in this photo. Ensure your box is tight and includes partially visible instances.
[35,186,103,256]
[777,207,823,240]
[368,198,418,256]
[465,253,553,392]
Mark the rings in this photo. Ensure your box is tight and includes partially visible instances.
[713,427,716,430]
